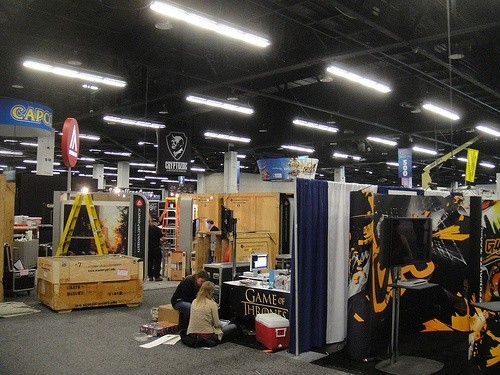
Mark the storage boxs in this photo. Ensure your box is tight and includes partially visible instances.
[5,242,36,292]
[36,280,144,312]
[37,254,143,284]
[255,313,289,351]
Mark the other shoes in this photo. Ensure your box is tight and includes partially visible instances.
[155,277,163,281]
[149,277,153,281]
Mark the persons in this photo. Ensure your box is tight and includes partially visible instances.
[148,210,162,282]
[206,219,220,264]
[170,270,209,340]
[186,281,236,346]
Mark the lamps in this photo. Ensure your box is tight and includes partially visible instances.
[448,43,465,59]
[159,104,168,114]
[326,114,336,123]
[410,106,421,112]
[68,50,83,65]
[150,0,272,47]
[23,56,128,87]
[11,77,24,88]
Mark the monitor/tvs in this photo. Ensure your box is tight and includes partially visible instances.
[221,205,233,240]
[379,217,431,268]
[249,253,269,271]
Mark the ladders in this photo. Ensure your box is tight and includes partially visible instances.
[55,192,109,257]
[161,197,180,277]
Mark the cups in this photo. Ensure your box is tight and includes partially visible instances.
[253,269,258,277]
